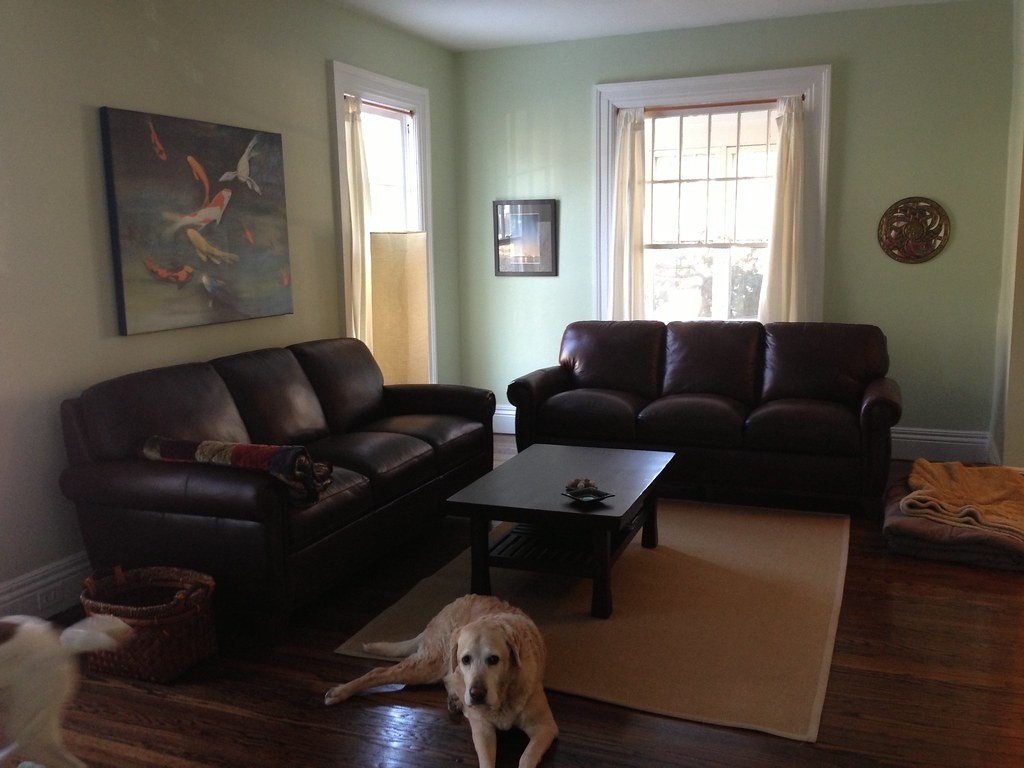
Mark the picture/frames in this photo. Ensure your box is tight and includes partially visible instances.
[494,199,557,276]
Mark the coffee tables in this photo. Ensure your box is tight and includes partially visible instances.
[444,443,679,619]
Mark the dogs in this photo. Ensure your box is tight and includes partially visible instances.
[325,594,559,768]
[0,613,136,767]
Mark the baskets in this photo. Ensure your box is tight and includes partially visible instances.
[79,564,219,686]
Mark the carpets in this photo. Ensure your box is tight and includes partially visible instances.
[335,498,853,743]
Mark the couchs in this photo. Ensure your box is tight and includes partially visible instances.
[505,320,905,514]
[59,337,496,630]
